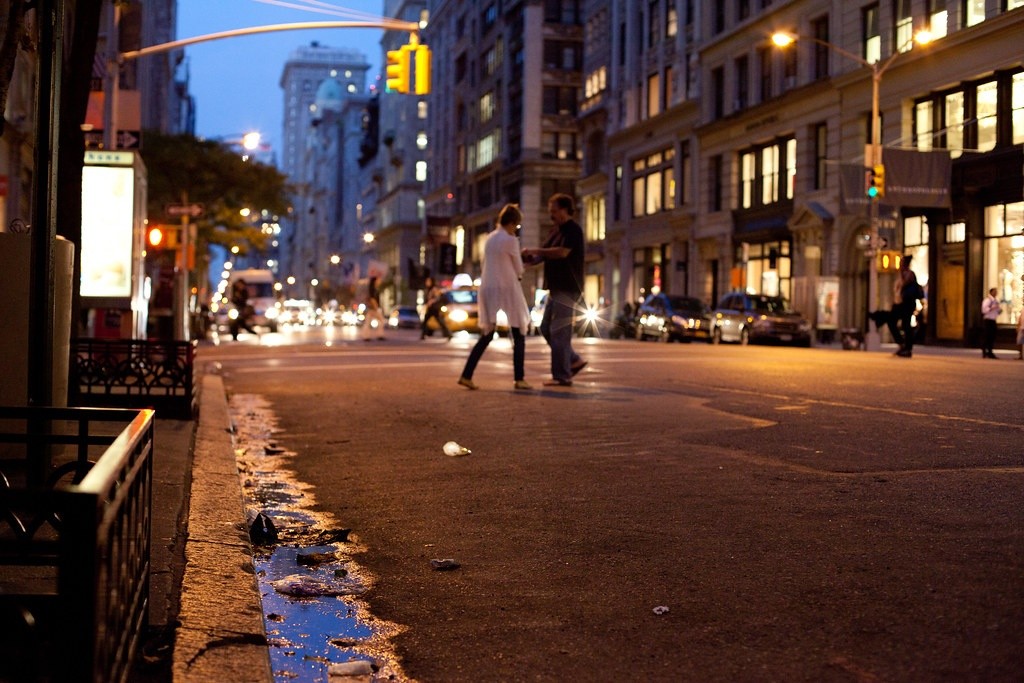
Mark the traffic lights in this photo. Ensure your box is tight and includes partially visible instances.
[147,225,177,248]
[387,45,410,94]
[868,164,885,199]
[876,250,901,272]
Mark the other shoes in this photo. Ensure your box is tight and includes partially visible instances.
[513,380,533,390]
[895,347,912,357]
[457,376,476,390]
[983,351,999,359]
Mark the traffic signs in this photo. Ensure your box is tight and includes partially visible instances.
[165,201,205,219]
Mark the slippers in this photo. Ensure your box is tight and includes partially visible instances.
[568,359,588,377]
[543,377,572,386]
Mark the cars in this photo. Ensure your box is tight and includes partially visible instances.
[636,294,709,343]
[214,308,227,325]
[423,286,510,338]
[710,293,812,346]
[390,305,420,328]
[280,302,384,328]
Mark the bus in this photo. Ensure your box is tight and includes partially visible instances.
[227,268,276,332]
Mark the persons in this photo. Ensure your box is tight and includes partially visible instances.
[981,289,1002,359]
[230,279,261,342]
[887,255,917,357]
[457,204,533,389]
[420,277,454,342]
[521,193,589,386]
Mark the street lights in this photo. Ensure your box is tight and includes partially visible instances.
[773,29,929,351]
